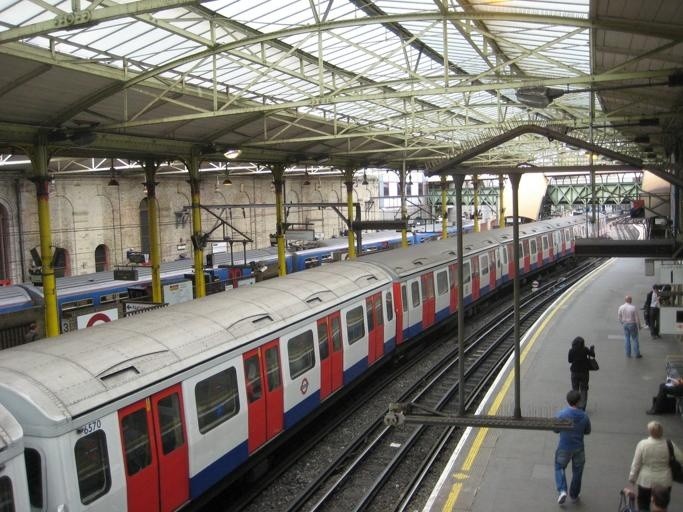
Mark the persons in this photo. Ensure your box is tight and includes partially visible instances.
[616,295,642,359]
[646,374,683,415]
[567,335,596,412]
[623,420,682,511]
[639,282,662,340]
[648,483,671,511]
[21,321,40,344]
[548,390,591,505]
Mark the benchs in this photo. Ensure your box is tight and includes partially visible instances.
[664,353,683,422]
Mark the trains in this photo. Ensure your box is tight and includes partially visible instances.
[0,207,612,512]
[0,209,500,348]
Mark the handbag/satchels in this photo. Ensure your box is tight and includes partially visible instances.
[586,349,599,371]
[643,309,650,326]
[652,391,677,413]
[665,438,683,485]
[618,489,635,512]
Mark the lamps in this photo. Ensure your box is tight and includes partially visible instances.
[515,73,683,108]
[200,146,242,160]
[407,171,413,186]
[545,117,659,136]
[303,165,311,186]
[47,129,98,147]
[289,154,331,166]
[362,168,368,185]
[223,161,232,186]
[107,158,120,187]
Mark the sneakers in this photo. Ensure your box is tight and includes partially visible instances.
[636,354,642,358]
[649,333,662,340]
[646,408,663,415]
[571,493,581,505]
[557,491,568,504]
